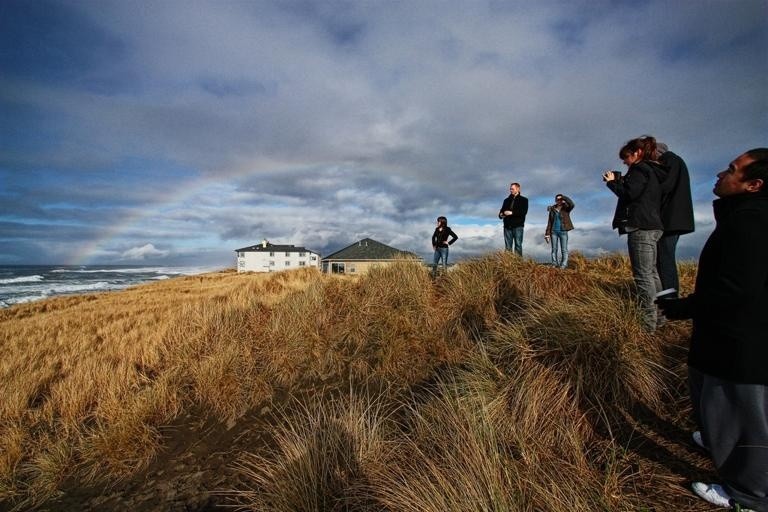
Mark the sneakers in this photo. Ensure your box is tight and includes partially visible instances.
[691,482,748,512]
[692,431,709,449]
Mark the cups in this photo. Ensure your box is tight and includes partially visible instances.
[654,286,678,321]
[546,237,550,244]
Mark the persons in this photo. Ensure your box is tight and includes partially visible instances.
[428,214,459,277]
[498,183,530,256]
[647,142,696,301]
[601,134,670,337]
[652,146,768,511]
[543,192,575,268]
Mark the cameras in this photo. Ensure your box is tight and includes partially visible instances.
[603,171,621,182]
